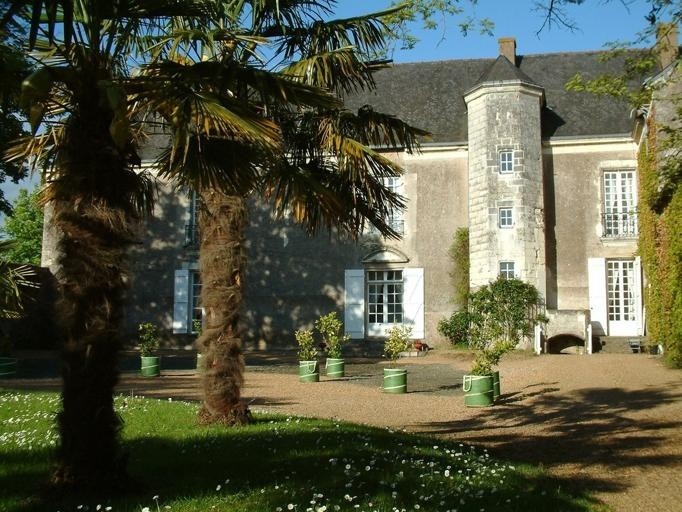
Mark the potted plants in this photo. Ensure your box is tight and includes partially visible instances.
[435,276,550,410]
[137,321,163,377]
[379,319,418,392]
[192,316,205,368]
[294,309,360,382]
